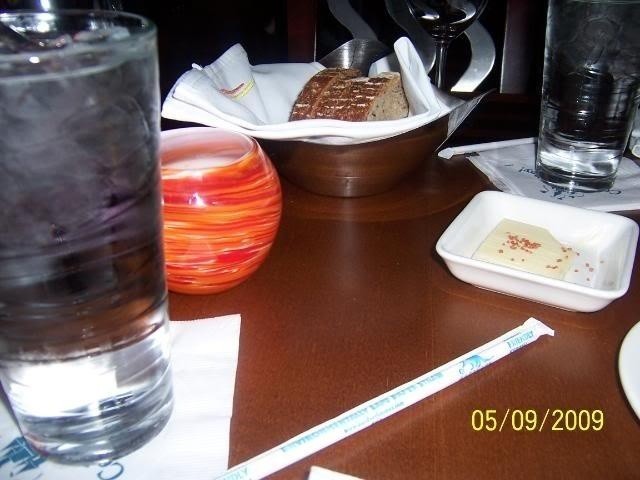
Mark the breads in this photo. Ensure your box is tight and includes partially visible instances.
[286,64,411,124]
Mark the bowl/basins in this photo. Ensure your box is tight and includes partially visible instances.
[254,33,489,199]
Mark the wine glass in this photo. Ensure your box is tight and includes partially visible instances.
[407,0,488,99]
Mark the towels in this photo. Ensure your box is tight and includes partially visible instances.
[161,37,465,148]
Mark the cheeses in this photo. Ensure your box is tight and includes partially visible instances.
[472,219,571,279]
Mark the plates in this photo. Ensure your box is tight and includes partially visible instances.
[615,322,640,421]
[435,190,639,317]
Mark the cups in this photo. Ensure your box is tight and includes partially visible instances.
[1,10,178,463]
[161,126,283,298]
[534,0,640,192]
[0,0,120,50]
